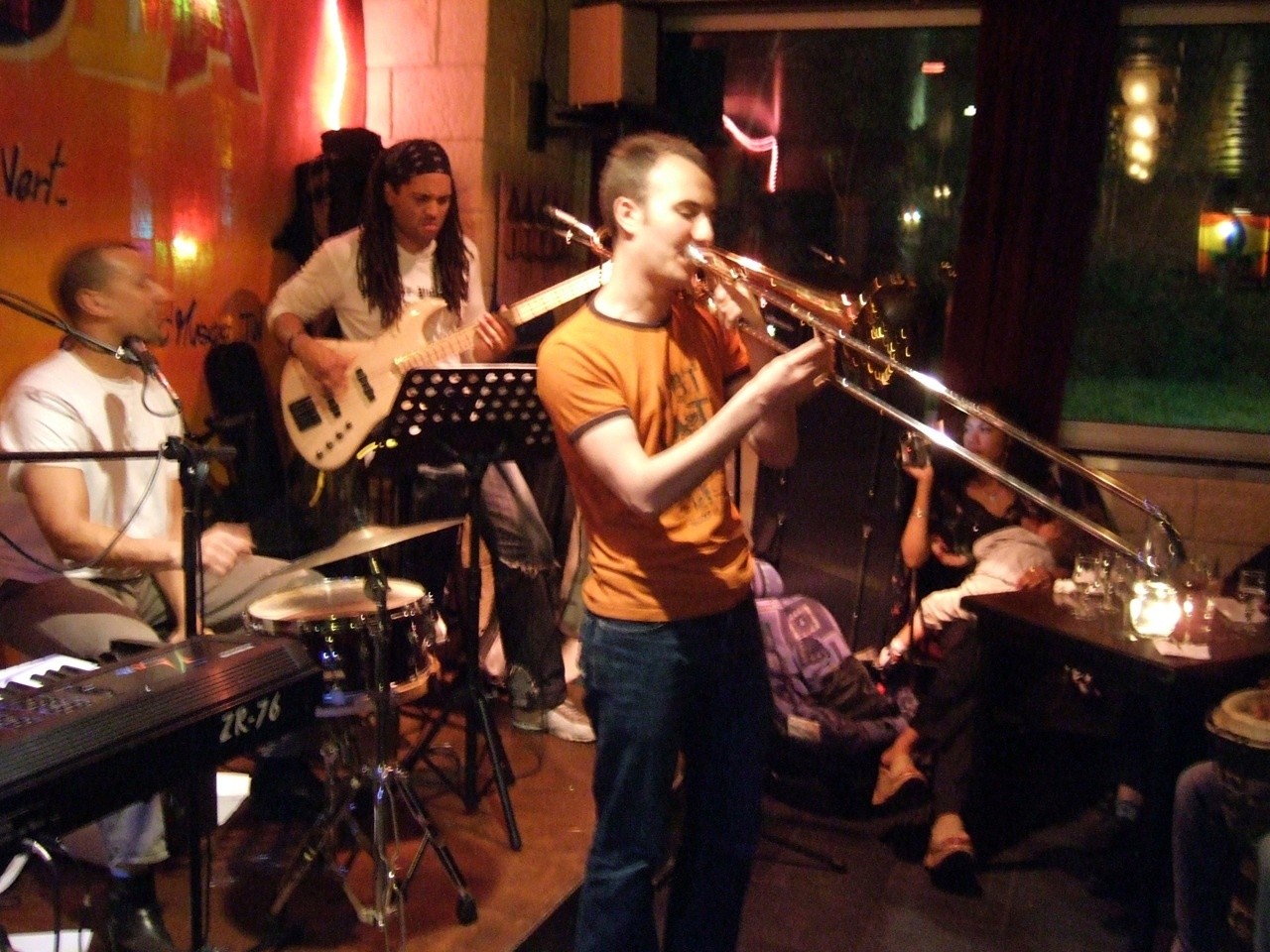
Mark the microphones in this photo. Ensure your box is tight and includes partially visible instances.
[123,334,187,415]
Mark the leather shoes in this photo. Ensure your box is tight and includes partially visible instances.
[104,905,177,952]
[253,756,363,818]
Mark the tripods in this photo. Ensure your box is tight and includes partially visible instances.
[402,444,524,853]
[847,330,916,658]
[259,552,478,948]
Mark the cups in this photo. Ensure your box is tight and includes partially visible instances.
[1073,535,1228,654]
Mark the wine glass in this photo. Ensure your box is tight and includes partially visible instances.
[1238,572,1267,638]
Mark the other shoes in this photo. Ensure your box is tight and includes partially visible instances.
[1115,799,1142,830]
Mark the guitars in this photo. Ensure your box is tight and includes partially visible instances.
[277,253,615,474]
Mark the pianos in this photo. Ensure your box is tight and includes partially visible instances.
[0,630,321,858]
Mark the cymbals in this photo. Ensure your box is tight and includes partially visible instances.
[259,513,467,589]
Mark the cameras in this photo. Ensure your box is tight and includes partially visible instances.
[896,419,945,468]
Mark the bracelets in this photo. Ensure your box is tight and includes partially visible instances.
[288,331,310,355]
[909,508,929,518]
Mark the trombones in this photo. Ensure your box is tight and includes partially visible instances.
[541,202,1186,574]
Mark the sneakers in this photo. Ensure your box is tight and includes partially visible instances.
[510,701,596,743]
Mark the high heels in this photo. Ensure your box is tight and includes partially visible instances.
[870,754,927,805]
[925,815,973,871]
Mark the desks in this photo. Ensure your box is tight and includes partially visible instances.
[959,576,1270,852]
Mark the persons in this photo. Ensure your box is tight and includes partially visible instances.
[3,236,344,952]
[264,138,596,743]
[876,510,1074,673]
[1168,762,1270,952]
[747,463,913,761]
[873,400,1077,878]
[532,132,836,952]
[1105,538,1270,852]
[546,503,596,703]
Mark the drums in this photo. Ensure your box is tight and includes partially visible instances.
[243,574,439,720]
